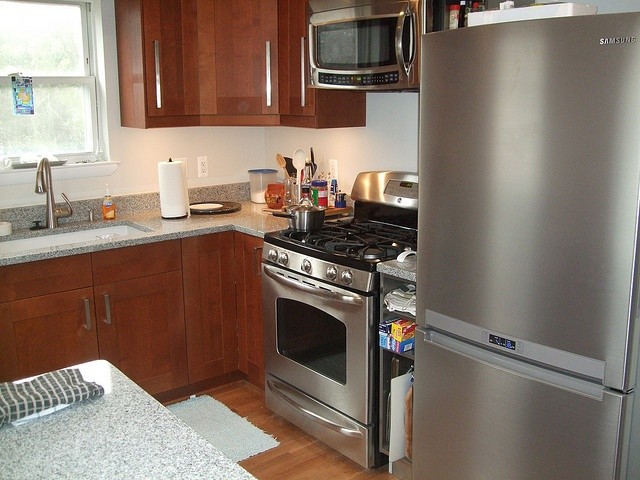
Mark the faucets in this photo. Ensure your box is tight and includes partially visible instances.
[34,157,74,231]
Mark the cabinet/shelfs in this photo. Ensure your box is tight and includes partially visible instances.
[1,236,189,400]
[378,260,416,463]
[232,224,267,391]
[112,1,367,131]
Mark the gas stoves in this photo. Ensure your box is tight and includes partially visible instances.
[262,172,418,295]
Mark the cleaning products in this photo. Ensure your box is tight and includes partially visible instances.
[102,182,115,222]
[101,182,116,220]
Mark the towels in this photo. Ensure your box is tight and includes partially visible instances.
[0,368,104,424]
[12,359,112,426]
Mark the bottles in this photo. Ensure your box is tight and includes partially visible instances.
[301,184,310,205]
[312,180,329,212]
[264,184,284,209]
[248,169,278,204]
[335,192,348,207]
[329,179,340,207]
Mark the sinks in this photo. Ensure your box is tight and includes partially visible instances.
[0,220,158,259]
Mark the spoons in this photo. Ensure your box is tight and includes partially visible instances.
[293,147,306,202]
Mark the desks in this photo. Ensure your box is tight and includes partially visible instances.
[1,358,255,479]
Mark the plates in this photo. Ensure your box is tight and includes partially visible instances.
[190,201,242,214]
[192,202,222,210]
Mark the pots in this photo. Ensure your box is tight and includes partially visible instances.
[272,208,326,232]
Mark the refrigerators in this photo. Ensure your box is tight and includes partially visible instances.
[413,14,640,480]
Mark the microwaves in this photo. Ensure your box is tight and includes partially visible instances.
[310,0,426,89]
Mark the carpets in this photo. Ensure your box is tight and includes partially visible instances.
[164,392,280,464]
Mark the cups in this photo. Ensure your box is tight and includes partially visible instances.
[285,180,302,211]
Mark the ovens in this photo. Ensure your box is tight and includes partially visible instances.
[259,261,383,468]
